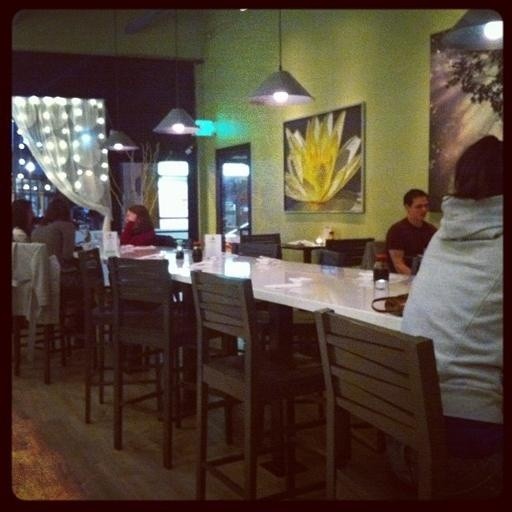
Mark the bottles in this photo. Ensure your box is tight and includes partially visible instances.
[176,240,184,260]
[373,254,389,290]
[192,242,202,262]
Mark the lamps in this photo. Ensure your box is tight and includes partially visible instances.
[98,10,141,152]
[152,9,200,136]
[436,9,503,50]
[248,9,313,107]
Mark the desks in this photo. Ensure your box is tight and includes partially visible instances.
[73,240,416,333]
[281,239,331,265]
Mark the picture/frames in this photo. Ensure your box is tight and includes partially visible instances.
[282,101,366,215]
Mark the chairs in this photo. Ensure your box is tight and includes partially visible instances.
[312,308,501,501]
[108,255,189,469]
[76,248,166,424]
[319,238,375,268]
[12,240,69,385]
[233,233,282,261]
[189,268,326,500]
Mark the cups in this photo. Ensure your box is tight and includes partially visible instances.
[103,231,120,254]
[204,234,222,258]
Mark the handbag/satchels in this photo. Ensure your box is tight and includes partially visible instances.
[371,293,408,316]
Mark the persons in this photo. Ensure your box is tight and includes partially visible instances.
[385,189,438,275]
[400,137,503,503]
[12,196,35,243]
[121,204,159,245]
[32,198,80,329]
